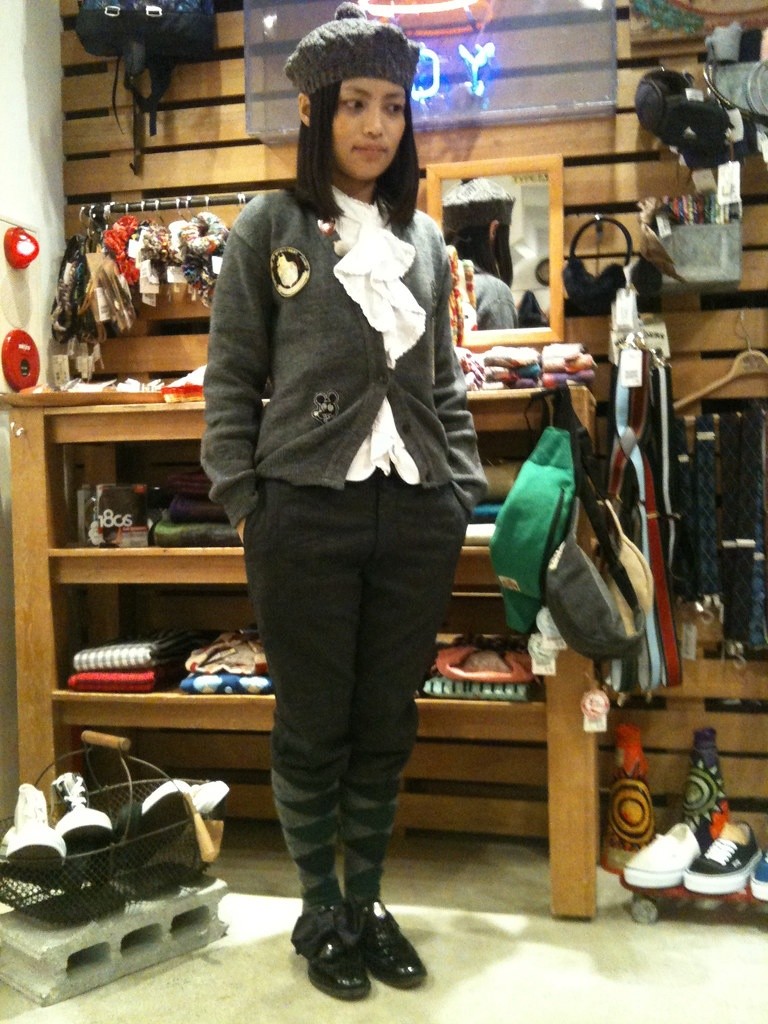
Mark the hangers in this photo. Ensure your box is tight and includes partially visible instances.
[669,307,768,418]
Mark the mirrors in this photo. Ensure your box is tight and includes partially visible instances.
[430,152,565,348]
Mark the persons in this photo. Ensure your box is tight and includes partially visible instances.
[199,17,490,1000]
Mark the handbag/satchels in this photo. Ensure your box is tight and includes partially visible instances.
[490,381,653,657]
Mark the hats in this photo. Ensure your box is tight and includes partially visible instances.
[442,177,517,233]
[283,1,420,96]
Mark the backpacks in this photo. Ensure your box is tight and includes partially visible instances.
[75,0,211,174]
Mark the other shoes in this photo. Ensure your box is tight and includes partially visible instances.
[750,853,768,900]
[117,780,231,869]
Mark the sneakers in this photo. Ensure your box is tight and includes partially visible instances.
[684,820,764,895]
[623,823,701,889]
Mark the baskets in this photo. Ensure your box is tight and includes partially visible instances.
[0,728,226,928]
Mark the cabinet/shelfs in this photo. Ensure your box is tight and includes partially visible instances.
[0,374,597,920]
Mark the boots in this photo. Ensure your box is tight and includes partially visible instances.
[51,772,113,846]
[2,782,66,871]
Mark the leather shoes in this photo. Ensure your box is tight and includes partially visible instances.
[346,898,427,987]
[291,900,370,1001]
